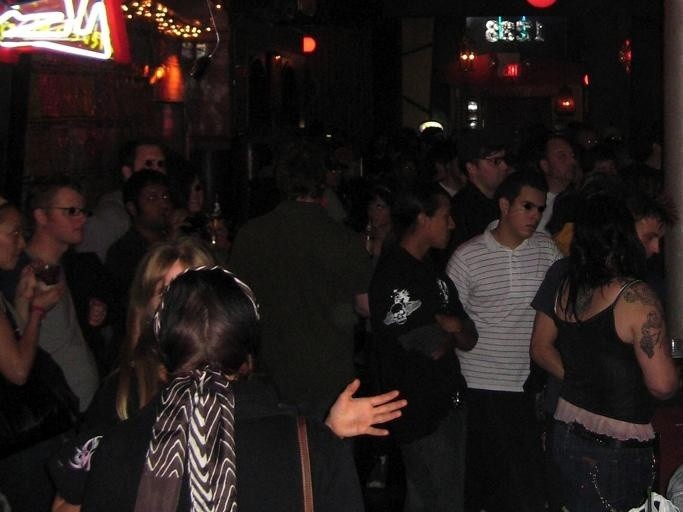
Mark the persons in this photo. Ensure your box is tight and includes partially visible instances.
[0,121,682,510]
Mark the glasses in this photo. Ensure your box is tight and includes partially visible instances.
[41,205,96,220]
[475,155,509,168]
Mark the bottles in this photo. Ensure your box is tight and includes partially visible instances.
[360,217,375,257]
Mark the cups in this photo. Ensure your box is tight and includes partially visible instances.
[668,337,682,358]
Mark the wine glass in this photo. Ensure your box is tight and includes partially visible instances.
[204,211,225,252]
